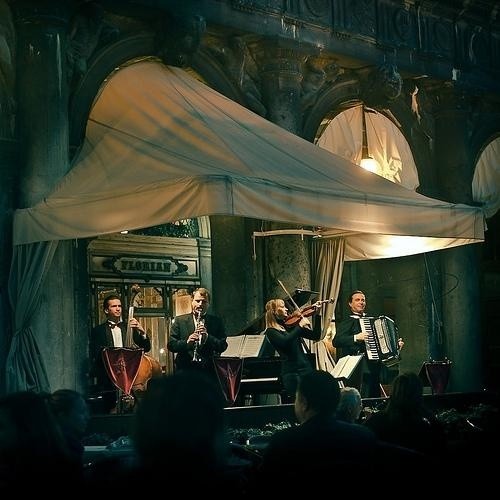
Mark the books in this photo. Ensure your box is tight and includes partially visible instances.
[220,334,266,359]
[330,354,362,379]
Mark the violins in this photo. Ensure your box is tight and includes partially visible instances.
[283,297,336,332]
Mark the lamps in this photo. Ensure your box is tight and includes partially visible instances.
[360,105,379,173]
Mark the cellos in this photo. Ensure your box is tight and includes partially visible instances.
[111,281,163,417]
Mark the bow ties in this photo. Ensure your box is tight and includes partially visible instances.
[111,322,121,328]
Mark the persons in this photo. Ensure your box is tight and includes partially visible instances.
[331,291,405,399]
[0,369,500,499]
[91,295,151,409]
[265,298,323,395]
[167,288,228,375]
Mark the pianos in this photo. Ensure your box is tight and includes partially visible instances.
[213,289,321,407]
